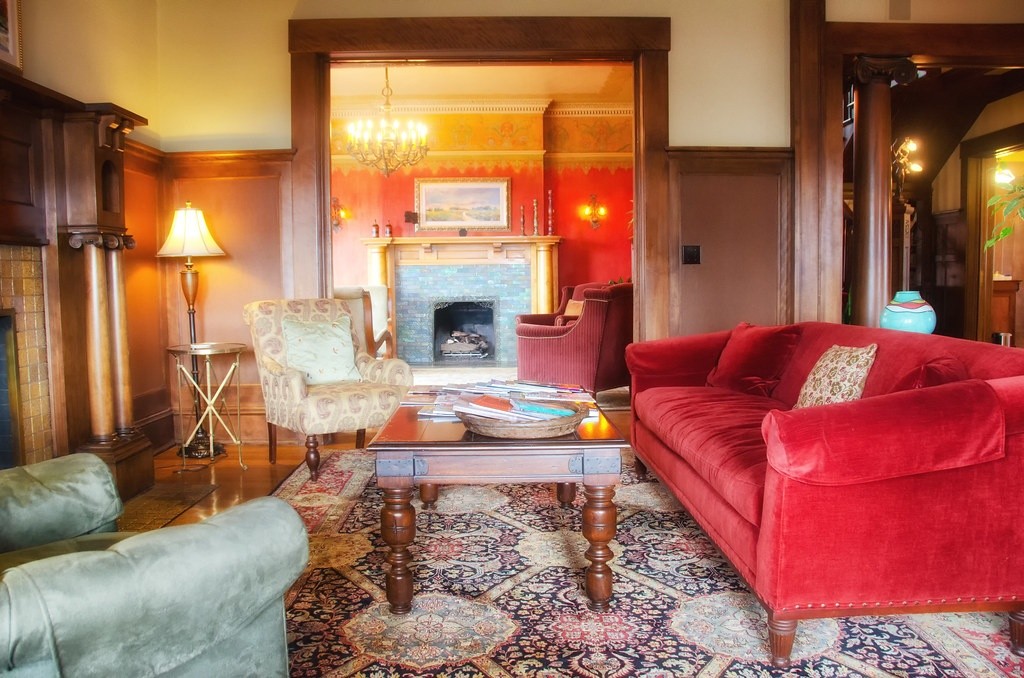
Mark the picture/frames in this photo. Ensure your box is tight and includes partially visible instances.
[412,177,513,232]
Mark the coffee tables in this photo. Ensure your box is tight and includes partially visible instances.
[368,379,630,612]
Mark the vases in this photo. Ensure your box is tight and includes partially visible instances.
[880,290,937,336]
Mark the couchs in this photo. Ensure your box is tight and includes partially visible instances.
[0,452,308,678]
[624,322,1024,671]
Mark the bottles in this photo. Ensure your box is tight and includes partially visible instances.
[386,219,392,237]
[373,219,379,238]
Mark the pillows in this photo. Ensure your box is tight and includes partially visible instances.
[706,322,803,396]
[280,314,364,386]
[793,342,878,411]
[887,348,966,393]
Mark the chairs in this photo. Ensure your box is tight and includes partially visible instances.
[333,287,394,360]
[248,296,414,481]
[516,277,634,397]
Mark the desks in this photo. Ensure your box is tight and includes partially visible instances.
[163,341,248,470]
[992,280,1023,347]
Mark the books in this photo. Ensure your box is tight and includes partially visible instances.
[402,378,599,423]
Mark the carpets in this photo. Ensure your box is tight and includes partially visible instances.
[264,448,1024,678]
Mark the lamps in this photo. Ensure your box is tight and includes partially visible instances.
[342,64,429,175]
[895,140,925,201]
[331,197,347,227]
[152,201,225,459]
[580,195,609,229]
